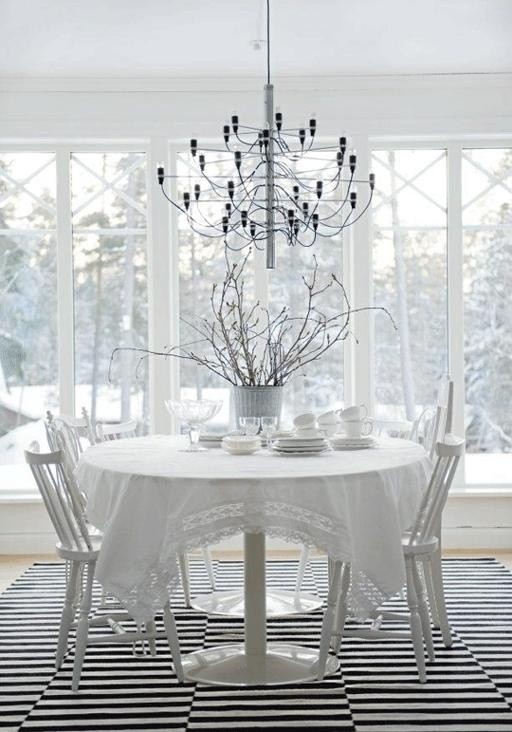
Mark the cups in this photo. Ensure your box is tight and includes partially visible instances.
[340,403,368,419]
[296,428,329,438]
[317,408,342,424]
[341,422,372,439]
[293,413,316,429]
[319,424,340,436]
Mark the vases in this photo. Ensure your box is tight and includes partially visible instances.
[230,386,282,440]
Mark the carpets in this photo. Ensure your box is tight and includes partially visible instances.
[0,558,511,731]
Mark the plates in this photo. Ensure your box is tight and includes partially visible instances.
[221,436,261,456]
[331,437,380,449]
[272,438,330,456]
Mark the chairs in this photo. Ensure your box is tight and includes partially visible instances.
[44,409,95,524]
[98,419,215,608]
[320,439,467,686]
[21,441,186,689]
[411,375,454,628]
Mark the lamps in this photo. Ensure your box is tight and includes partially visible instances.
[156,0,377,269]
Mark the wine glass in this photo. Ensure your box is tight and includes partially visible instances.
[239,416,255,433]
[261,417,278,453]
[162,398,225,452]
[244,418,260,437]
